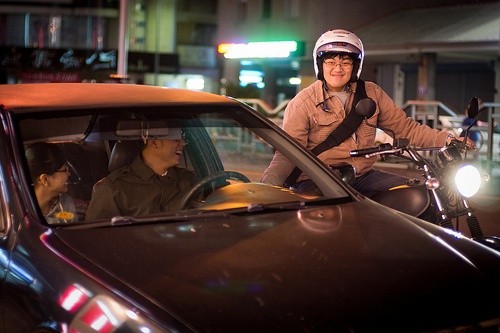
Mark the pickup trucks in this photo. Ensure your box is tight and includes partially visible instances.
[407,104,499,156]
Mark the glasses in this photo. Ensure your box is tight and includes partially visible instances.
[323,60,354,67]
[52,166,69,173]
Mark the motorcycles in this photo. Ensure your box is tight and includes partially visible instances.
[349,135,500,252]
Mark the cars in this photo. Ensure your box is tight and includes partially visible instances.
[0,83,499,332]
[374,115,500,169]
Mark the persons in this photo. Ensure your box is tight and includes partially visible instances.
[84,122,245,222]
[259,29,478,198]
[22,141,78,223]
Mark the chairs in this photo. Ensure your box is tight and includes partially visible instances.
[107,139,146,171]
[65,141,110,201]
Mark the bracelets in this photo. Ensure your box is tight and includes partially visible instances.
[447,134,454,139]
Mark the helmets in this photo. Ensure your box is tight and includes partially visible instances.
[311,28,364,82]
